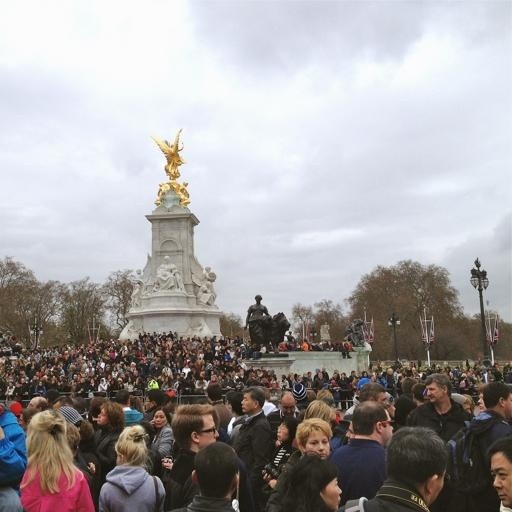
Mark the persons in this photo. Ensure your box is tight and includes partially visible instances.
[198,282,216,305]
[203,267,217,287]
[151,256,182,289]
[0,331,511,512]
[244,294,270,329]
[130,269,143,306]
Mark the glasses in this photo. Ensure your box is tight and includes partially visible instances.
[75,421,81,427]
[202,426,216,434]
[381,420,396,426]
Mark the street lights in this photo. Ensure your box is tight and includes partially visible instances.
[309,326,321,347]
[388,307,405,358]
[467,254,498,365]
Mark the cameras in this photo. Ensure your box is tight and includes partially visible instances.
[266,463,280,479]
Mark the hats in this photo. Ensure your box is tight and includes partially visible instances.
[292,382,307,401]
[58,406,82,424]
[356,377,371,389]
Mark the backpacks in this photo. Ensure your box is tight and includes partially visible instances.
[441,415,505,497]
